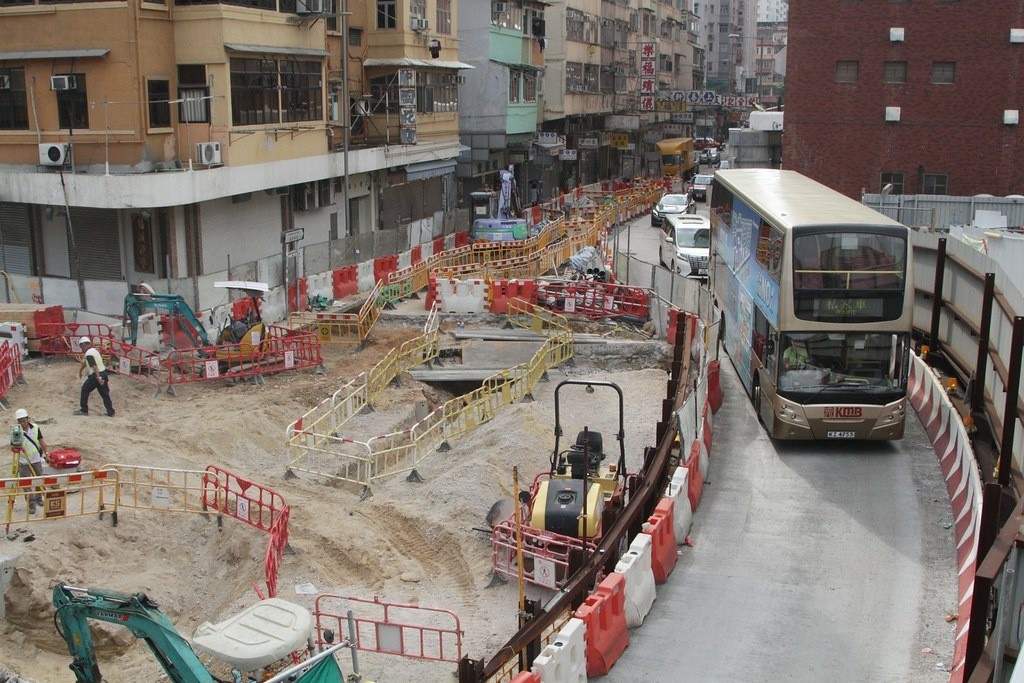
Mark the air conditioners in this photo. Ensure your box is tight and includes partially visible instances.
[412,18,428,28]
[356,100,370,113]
[296,0,324,13]
[0,74,11,90]
[38,141,70,167]
[195,142,221,164]
[459,75,467,83]
[50,74,78,91]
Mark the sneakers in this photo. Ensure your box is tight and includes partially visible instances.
[30,505,36,514]
[35,498,44,506]
[74,408,88,416]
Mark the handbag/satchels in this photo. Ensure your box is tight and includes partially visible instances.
[39,447,43,457]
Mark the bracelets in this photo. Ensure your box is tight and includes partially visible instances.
[45,451,48,454]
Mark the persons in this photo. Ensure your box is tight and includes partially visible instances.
[783,335,809,370]
[11,408,49,513]
[73,336,115,416]
[586,267,607,283]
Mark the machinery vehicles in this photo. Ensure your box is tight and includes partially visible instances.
[52,581,316,683]
[122,281,285,385]
[490,377,629,590]
[468,165,528,243]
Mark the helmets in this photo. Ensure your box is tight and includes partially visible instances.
[16,409,28,420]
[79,337,90,344]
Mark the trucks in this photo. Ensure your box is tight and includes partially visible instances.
[655,138,694,180]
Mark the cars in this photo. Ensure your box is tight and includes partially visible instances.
[693,137,726,152]
[687,174,714,201]
[700,148,721,163]
[651,192,697,227]
[659,214,711,278]
[715,161,729,170]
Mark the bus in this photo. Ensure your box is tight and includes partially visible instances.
[709,167,916,442]
